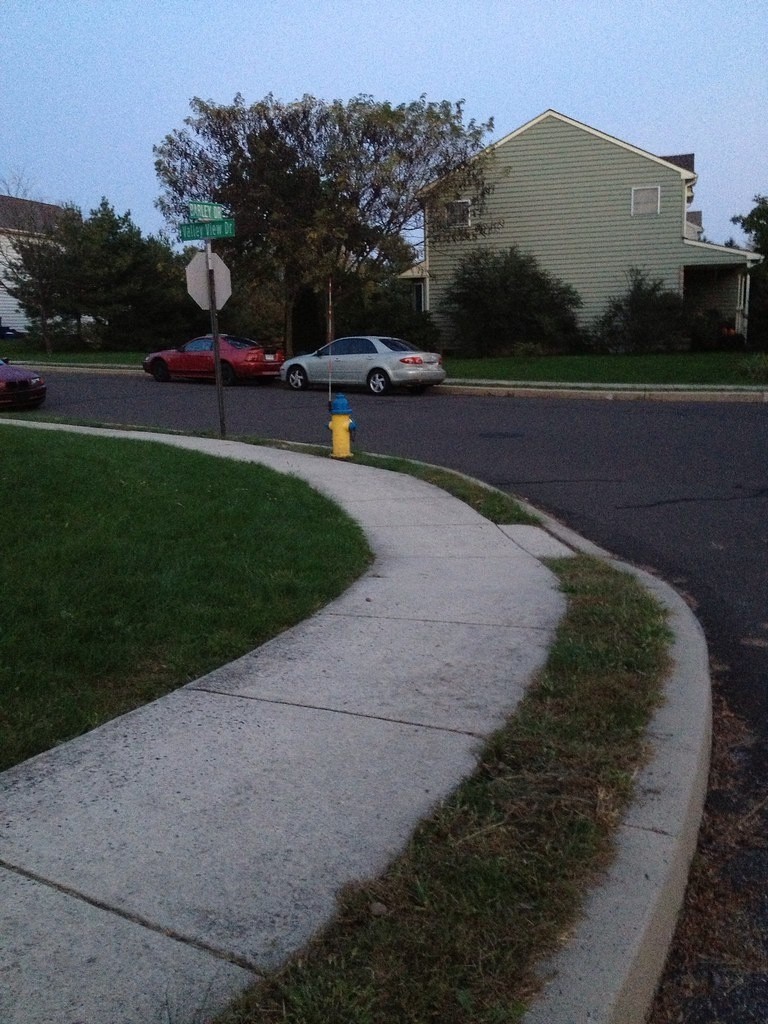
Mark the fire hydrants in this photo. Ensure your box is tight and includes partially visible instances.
[324,392,357,460]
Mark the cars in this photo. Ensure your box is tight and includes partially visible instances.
[0,355,47,412]
[141,333,288,386]
[280,336,447,396]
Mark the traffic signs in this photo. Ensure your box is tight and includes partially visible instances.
[189,201,222,220]
[179,218,236,241]
